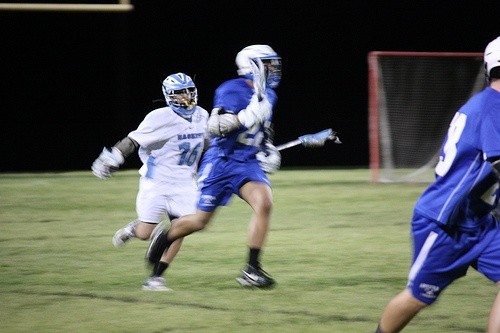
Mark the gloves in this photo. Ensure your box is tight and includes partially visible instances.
[255,144,282,175]
[91,146,120,180]
[238,91,273,129]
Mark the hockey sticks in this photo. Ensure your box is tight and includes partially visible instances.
[275,129,338,150]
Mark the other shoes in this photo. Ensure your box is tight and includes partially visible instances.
[142,274,176,292]
[145,220,172,265]
[236,263,276,290]
[112,219,139,249]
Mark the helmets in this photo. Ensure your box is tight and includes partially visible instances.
[483,36,500,80]
[235,44,282,88]
[162,72,198,119]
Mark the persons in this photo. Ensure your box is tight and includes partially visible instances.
[92,72,209,291]
[147,44,281,289]
[374,37,500,333]
